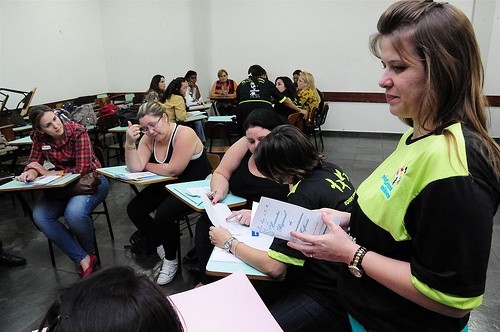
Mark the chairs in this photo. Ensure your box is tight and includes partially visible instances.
[48,97,329,267]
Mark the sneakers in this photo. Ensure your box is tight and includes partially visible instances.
[156,243,165,260]
[156,256,179,285]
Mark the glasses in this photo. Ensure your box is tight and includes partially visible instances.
[141,114,165,134]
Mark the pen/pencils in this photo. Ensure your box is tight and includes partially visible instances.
[196,190,217,207]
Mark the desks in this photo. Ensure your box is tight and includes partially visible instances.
[0,174,82,231]
[95,165,178,194]
[206,212,274,281]
[108,127,128,162]
[165,181,247,213]
[207,115,233,152]
[189,103,213,110]
[8,116,33,145]
[185,112,207,123]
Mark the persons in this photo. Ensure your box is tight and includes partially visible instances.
[287,1,500,332]
[39,262,187,332]
[13,105,110,279]
[124,64,355,332]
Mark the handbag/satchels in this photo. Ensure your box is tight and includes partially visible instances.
[183,247,201,271]
[43,171,101,200]
[54,102,117,129]
[0,134,11,156]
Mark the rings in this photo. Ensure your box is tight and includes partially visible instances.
[134,132,137,136]
[309,252,313,256]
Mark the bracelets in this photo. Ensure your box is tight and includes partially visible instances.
[233,241,244,257]
[124,143,135,151]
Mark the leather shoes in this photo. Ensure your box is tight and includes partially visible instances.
[0,251,25,265]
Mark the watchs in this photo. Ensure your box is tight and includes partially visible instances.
[347,246,370,277]
[224,236,237,253]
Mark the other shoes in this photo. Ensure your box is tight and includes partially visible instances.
[78,254,97,278]
